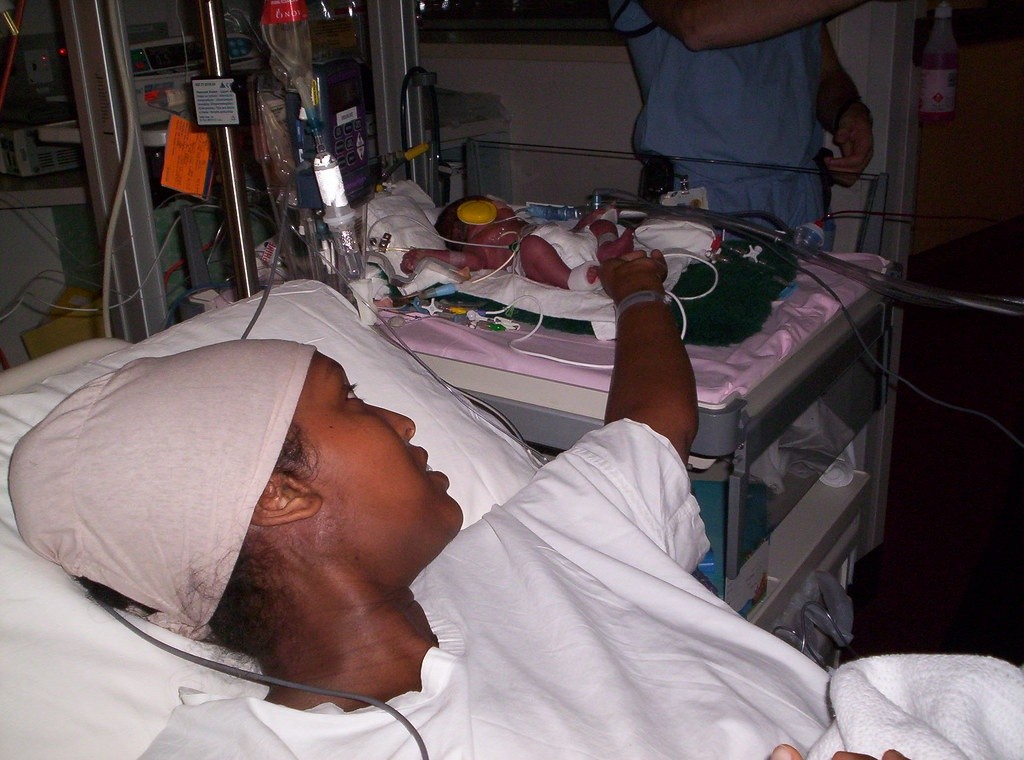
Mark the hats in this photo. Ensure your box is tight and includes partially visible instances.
[7,340,318,639]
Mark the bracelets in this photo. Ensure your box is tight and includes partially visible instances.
[614,290,673,324]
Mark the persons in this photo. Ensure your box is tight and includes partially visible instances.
[610,0,874,251]
[400,195,637,292]
[9,249,910,760]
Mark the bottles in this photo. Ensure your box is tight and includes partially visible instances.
[918,0,960,120]
